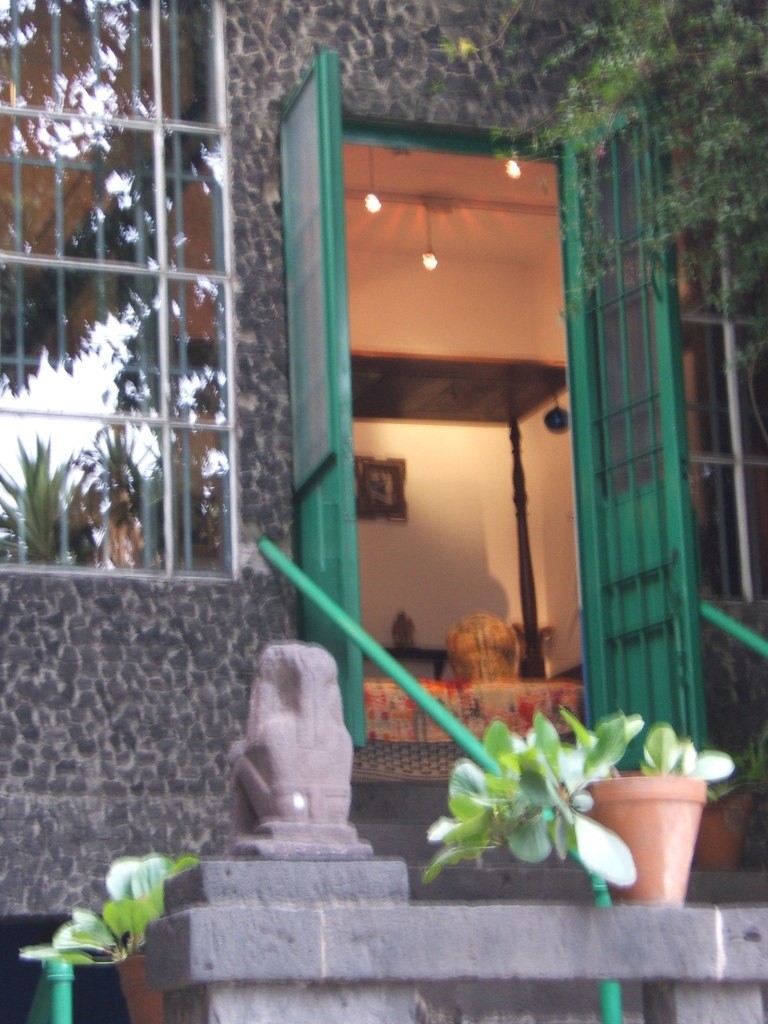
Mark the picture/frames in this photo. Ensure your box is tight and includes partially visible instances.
[356,456,408,521]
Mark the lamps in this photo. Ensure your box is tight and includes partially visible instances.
[422,205,437,270]
[366,148,381,213]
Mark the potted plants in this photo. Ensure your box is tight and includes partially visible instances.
[422,708,736,909]
[19,852,199,1024]
[693,727,768,873]
